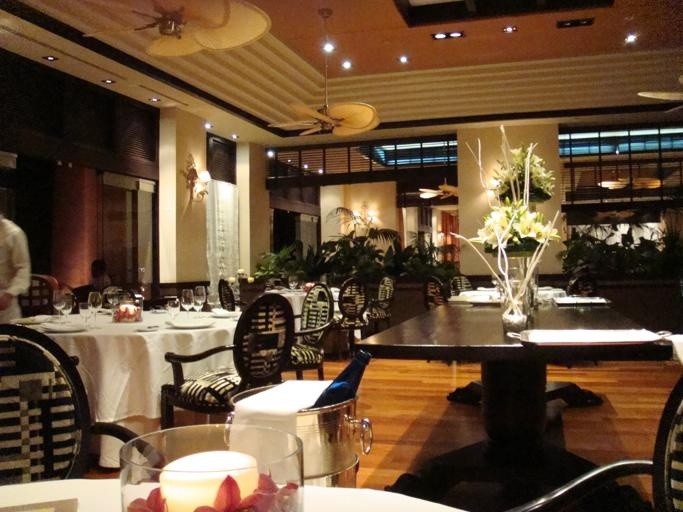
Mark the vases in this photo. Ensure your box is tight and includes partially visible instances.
[527,198,539,311]
[498,256,535,322]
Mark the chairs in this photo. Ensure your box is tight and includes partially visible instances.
[18,276,54,318]
[159,292,295,432]
[369,273,396,321]
[0,322,166,486]
[293,281,334,379]
[447,276,472,297]
[423,275,449,310]
[218,278,236,311]
[334,277,368,356]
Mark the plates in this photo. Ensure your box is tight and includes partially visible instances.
[40,321,87,333]
[212,305,241,319]
[136,325,159,333]
[16,315,54,324]
[165,316,215,329]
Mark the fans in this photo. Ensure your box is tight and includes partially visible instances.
[636,75,683,116]
[266,53,382,136]
[79,1,272,59]
[417,176,459,201]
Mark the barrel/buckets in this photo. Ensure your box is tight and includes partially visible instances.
[224,382,373,492]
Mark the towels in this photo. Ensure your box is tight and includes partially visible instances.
[134,323,162,332]
[554,296,608,304]
[538,288,565,294]
[534,286,553,290]
[459,290,501,301]
[518,327,662,342]
[97,308,113,316]
[151,308,168,314]
[660,332,682,364]
[35,314,52,322]
[269,289,286,294]
[229,379,333,450]
[330,286,340,292]
[477,287,497,291]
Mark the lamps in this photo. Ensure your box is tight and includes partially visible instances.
[182,152,213,204]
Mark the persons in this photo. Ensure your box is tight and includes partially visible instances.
[0,211,31,322]
[90,259,111,293]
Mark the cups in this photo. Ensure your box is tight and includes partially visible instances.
[118,423,306,512]
[167,300,181,317]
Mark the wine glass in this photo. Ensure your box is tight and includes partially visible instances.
[87,291,103,329]
[192,296,203,314]
[78,302,93,331]
[52,288,66,324]
[194,286,207,315]
[181,288,193,317]
[287,274,298,295]
[62,294,74,323]
[106,287,119,321]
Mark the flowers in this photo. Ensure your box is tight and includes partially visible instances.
[468,196,562,258]
[486,140,556,203]
